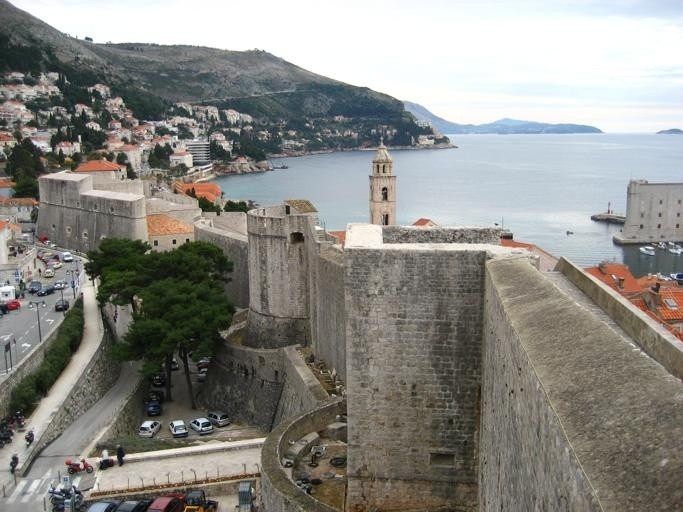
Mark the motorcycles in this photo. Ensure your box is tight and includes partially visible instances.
[0,409,34,474]
[48,483,86,511]
[65,456,93,473]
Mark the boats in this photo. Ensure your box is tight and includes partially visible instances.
[638,241,682,285]
[272,165,288,169]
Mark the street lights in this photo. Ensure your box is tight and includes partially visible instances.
[71,258,81,270]
[64,269,78,299]
[28,300,46,342]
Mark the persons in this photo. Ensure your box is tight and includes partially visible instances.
[114,443,125,467]
[97,445,109,470]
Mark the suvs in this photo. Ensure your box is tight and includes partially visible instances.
[189,416,213,435]
[205,410,230,427]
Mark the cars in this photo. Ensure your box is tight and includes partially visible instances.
[54,299,68,311]
[188,350,212,380]
[84,491,186,511]
[21,224,36,234]
[26,233,73,296]
[143,357,178,416]
[168,419,187,438]
[138,419,160,437]
[0,299,20,318]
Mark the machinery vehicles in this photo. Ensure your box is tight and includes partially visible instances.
[182,490,217,512]
